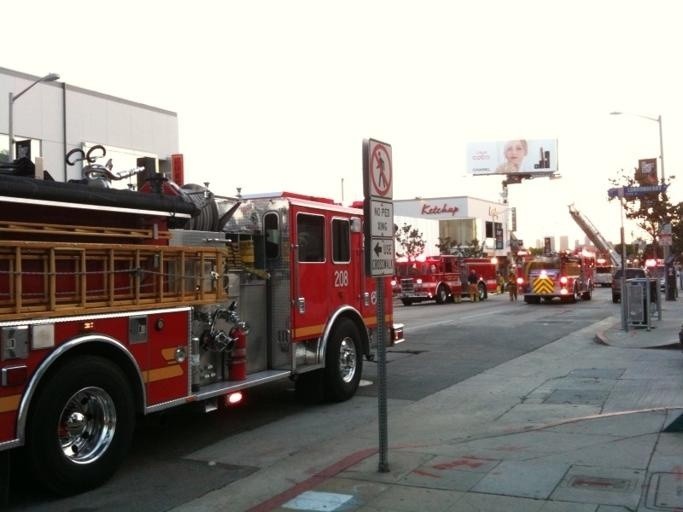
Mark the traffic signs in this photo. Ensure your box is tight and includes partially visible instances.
[369,197,396,278]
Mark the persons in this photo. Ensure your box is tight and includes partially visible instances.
[507,268,518,301]
[467,269,480,303]
[495,271,504,294]
[494,139,533,173]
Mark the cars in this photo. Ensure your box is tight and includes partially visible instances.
[611,267,647,304]
[644,265,668,293]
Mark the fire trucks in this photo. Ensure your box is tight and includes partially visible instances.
[516,249,595,306]
[396,253,498,308]
[567,202,622,288]
[0,143,406,493]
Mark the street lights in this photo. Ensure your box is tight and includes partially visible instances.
[609,110,671,301]
[8,71,60,171]
